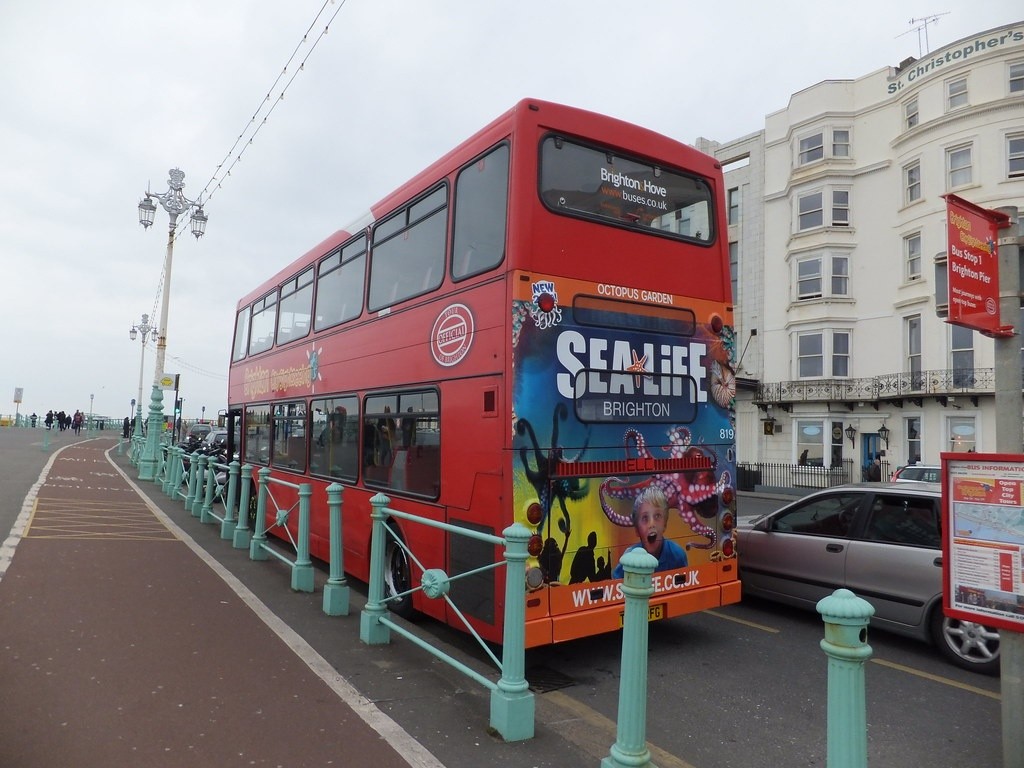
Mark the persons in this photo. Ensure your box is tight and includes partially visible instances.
[378,406,395,465]
[73,410,85,436]
[613,487,687,579]
[401,407,416,464]
[30,413,37,428]
[862,459,881,482]
[319,406,349,471]
[177,417,188,442]
[44,410,72,431]
[123,417,148,438]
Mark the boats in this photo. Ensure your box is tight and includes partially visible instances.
[80,413,109,427]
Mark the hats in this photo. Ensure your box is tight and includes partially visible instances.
[335,406,347,414]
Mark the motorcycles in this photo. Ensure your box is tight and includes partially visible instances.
[177,434,240,503]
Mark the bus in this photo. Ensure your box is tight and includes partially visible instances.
[225,98,743,651]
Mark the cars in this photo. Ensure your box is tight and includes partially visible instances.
[185,423,213,444]
[738,483,1001,674]
[201,430,238,449]
[891,464,942,485]
[287,424,304,438]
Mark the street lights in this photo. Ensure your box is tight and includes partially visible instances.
[201,406,205,423]
[128,325,158,461]
[88,394,94,438]
[139,193,207,482]
[129,399,136,443]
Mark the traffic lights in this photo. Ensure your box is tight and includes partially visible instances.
[176,401,180,413]
